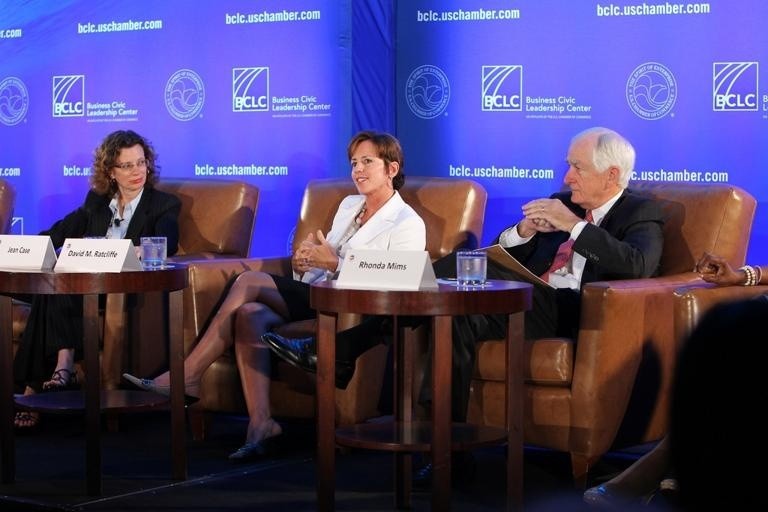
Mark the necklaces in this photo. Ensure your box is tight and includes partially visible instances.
[343,204,366,242]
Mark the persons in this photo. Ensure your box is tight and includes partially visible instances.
[122,130,426,461]
[260,127,665,494]
[12,131,182,434]
[582,249,768,512]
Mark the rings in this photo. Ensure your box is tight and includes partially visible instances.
[542,206,545,212]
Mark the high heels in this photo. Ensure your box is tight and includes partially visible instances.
[227,419,286,462]
[581,476,662,511]
[123,373,201,415]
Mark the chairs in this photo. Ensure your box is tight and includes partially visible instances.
[0,177,32,394]
[13,180,260,391]
[180,176,490,441]
[671,283,764,354]
[465,185,759,495]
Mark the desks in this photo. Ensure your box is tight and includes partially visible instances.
[308,278,534,512]
[0,262,190,495]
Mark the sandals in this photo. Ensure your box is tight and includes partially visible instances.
[12,391,44,433]
[41,368,86,392]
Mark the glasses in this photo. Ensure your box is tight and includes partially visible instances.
[112,159,150,170]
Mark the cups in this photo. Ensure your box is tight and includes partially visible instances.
[457,251,487,287]
[141,236,167,269]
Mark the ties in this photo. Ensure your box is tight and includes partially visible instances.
[539,210,595,284]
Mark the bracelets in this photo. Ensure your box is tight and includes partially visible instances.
[738,264,763,286]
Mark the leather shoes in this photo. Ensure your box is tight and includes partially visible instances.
[261,331,358,390]
[413,448,467,490]
